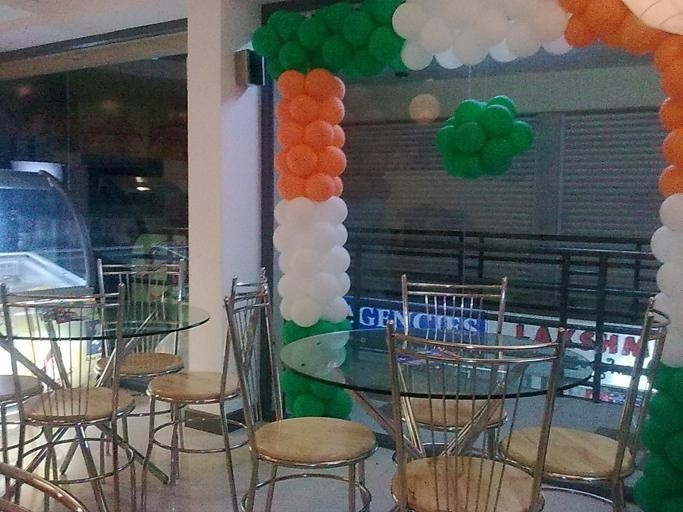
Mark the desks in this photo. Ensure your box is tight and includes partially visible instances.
[1,302,211,500]
[279,328,594,510]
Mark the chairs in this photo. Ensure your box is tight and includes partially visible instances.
[0,255,670,512]
